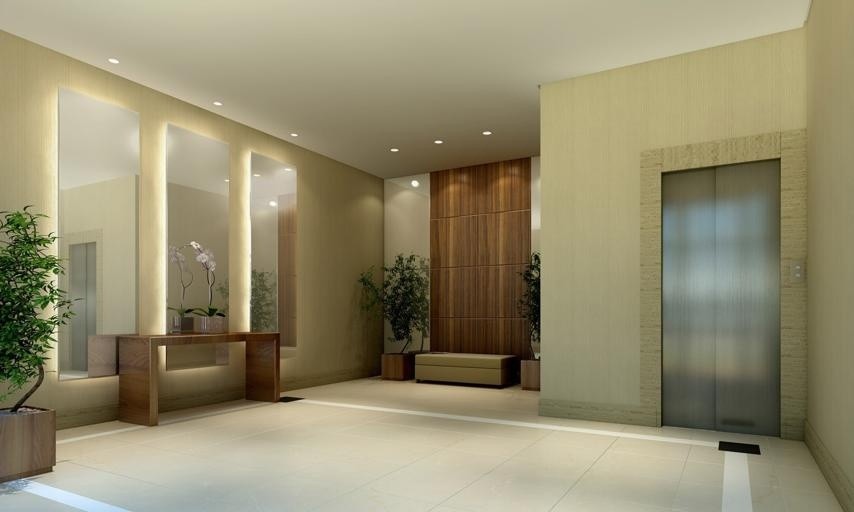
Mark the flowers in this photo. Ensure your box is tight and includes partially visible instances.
[165,237,201,315]
[188,237,229,319]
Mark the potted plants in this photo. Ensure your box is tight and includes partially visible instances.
[357,249,429,383]
[0,199,89,483]
[510,249,542,391]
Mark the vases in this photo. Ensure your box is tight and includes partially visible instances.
[191,311,230,337]
[169,311,192,334]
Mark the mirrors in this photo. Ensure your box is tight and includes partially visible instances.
[246,144,301,362]
[53,81,142,382]
[161,119,235,373]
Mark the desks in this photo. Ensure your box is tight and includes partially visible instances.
[113,325,281,426]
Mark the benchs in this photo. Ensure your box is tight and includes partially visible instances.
[412,351,515,388]
